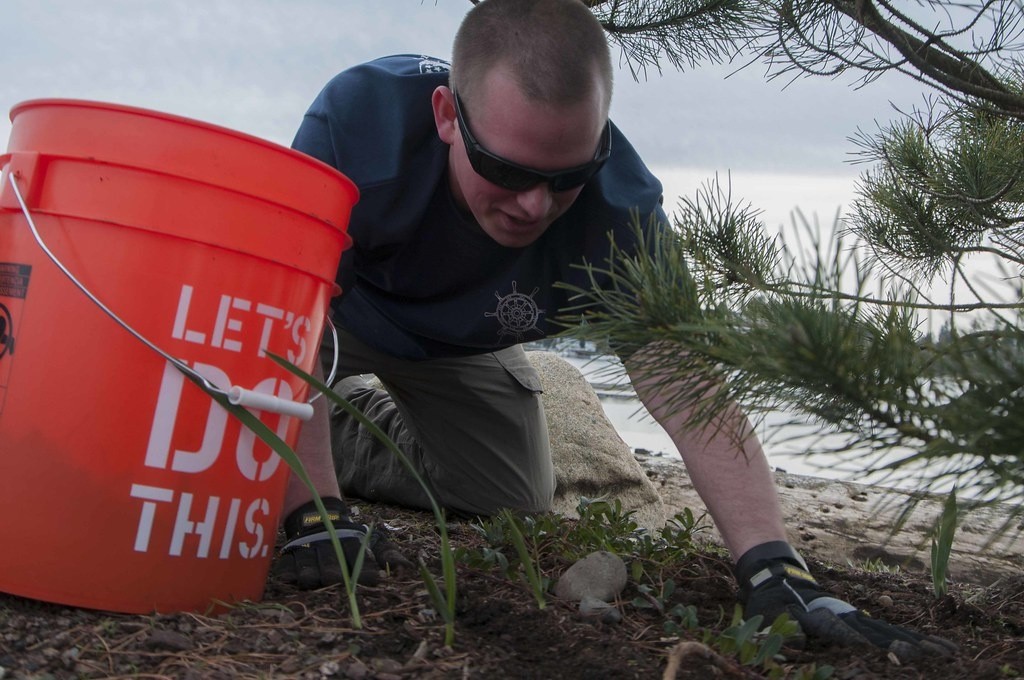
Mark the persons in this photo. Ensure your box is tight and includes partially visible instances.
[280,0,961,663]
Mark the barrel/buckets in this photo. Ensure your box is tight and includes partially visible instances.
[0,97,362,615]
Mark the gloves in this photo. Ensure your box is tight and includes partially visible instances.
[277,497,416,593]
[734,540,957,665]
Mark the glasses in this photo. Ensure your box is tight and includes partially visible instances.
[453,84,613,193]
